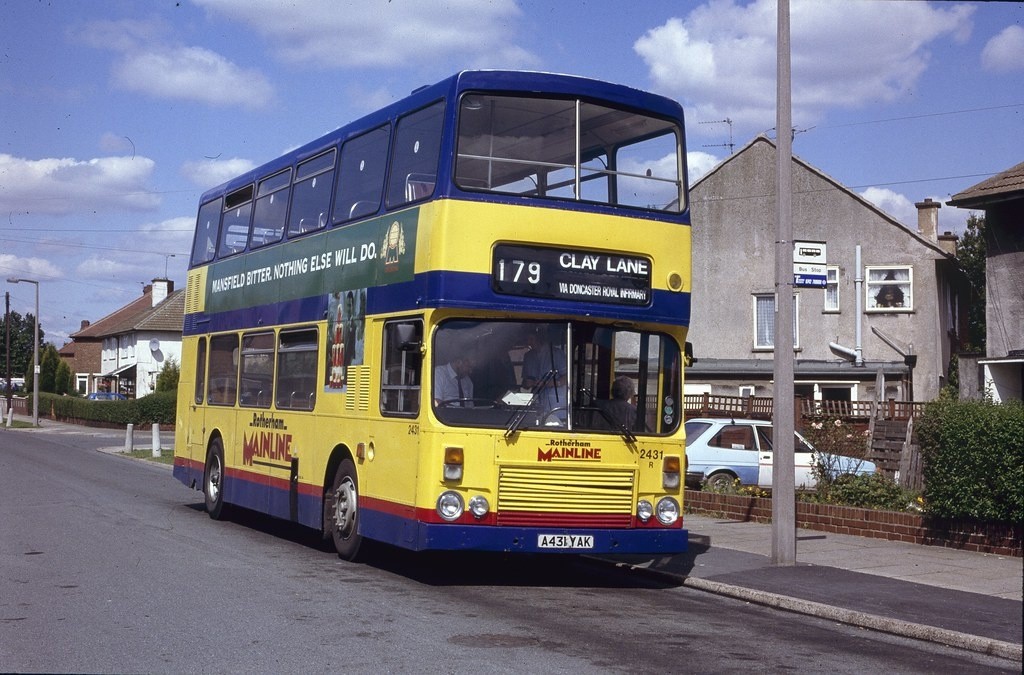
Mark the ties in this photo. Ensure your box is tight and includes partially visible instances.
[455,376,465,407]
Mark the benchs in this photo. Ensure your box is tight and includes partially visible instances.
[225,173,490,255]
[240,391,316,409]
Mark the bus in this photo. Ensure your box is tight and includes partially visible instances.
[170,66,697,567]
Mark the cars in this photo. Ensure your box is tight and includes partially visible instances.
[83,392,127,401]
[684,417,878,496]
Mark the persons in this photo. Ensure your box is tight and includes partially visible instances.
[430,350,475,410]
[522,326,568,426]
[580,378,639,431]
[346,292,357,365]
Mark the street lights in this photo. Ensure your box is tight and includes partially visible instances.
[6,276,40,427]
